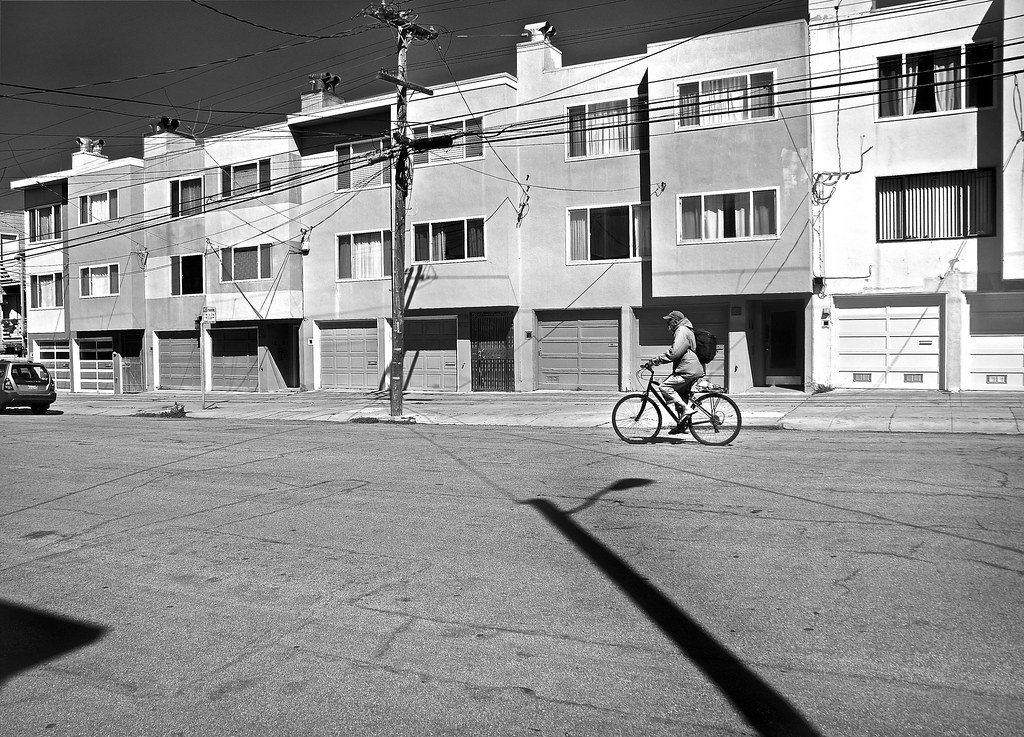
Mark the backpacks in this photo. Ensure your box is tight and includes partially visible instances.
[686,326,717,364]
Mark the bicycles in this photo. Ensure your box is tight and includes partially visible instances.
[612,356,742,447]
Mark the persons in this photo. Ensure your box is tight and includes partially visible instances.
[649,311,706,434]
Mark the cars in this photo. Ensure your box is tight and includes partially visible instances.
[0,361,57,415]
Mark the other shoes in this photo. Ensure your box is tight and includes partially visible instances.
[682,407,698,416]
[668,427,686,434]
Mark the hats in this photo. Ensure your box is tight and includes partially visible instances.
[663,311,684,319]
